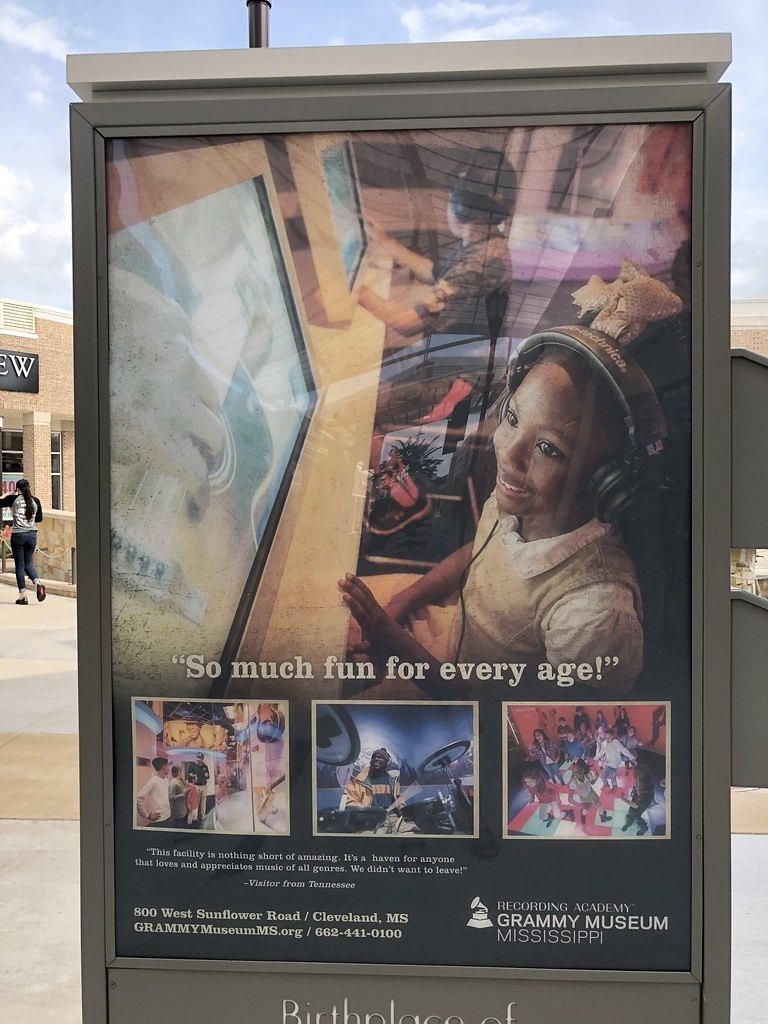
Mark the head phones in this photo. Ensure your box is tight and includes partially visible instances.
[497,324,673,531]
[451,147,488,222]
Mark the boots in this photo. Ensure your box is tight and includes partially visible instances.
[622,815,634,831]
[599,811,612,823]
[543,813,555,828]
[636,818,648,835]
[581,808,586,824]
[561,808,575,822]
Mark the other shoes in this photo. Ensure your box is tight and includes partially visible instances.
[624,768,629,776]
[553,783,567,793]
[37,584,46,602]
[600,784,610,790]
[187,820,200,828]
[16,597,28,604]
[630,763,636,770]
[608,788,617,794]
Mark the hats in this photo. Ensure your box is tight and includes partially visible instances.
[182,717,204,725]
[196,753,204,758]
[373,748,390,762]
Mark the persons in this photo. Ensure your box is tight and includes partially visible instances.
[0,479,46,604]
[357,146,518,535]
[522,707,667,838]
[338,324,675,735]
[138,752,211,828]
[346,748,424,837]
[179,720,206,748]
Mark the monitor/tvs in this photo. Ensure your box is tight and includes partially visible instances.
[105,141,329,698]
[281,131,374,323]
